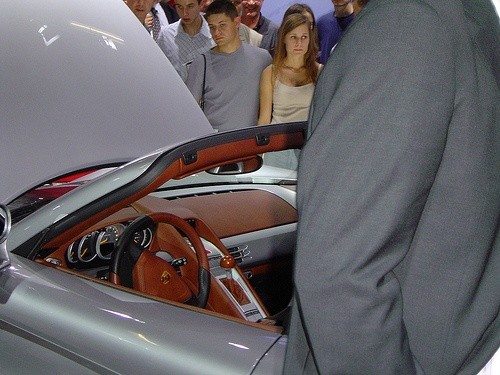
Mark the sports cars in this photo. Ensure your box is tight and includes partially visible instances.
[0,0,309,375]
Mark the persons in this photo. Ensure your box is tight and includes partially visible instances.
[258,13,327,126]
[184,0,274,134]
[283,1,500,375]
[275,3,324,64]
[314,0,364,70]
[156,0,217,84]
[233,0,282,61]
[124,0,278,56]
